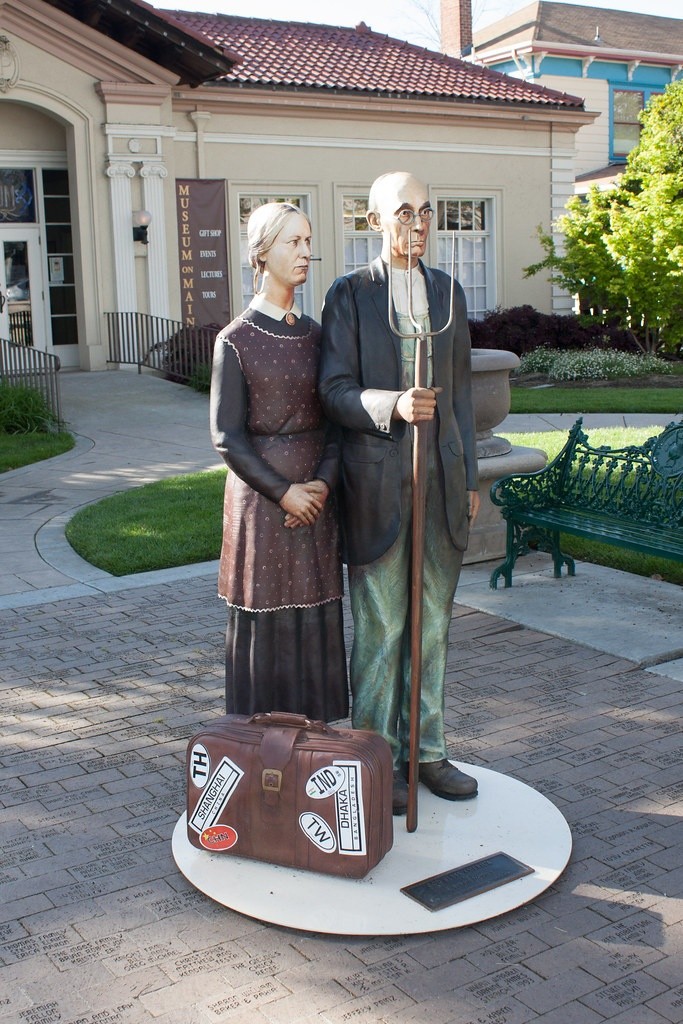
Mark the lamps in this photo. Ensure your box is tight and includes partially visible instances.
[133,210,152,244]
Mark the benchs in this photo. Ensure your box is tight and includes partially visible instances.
[489,416,683,590]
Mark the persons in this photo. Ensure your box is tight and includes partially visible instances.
[210,202,349,724]
[317,172,480,815]
